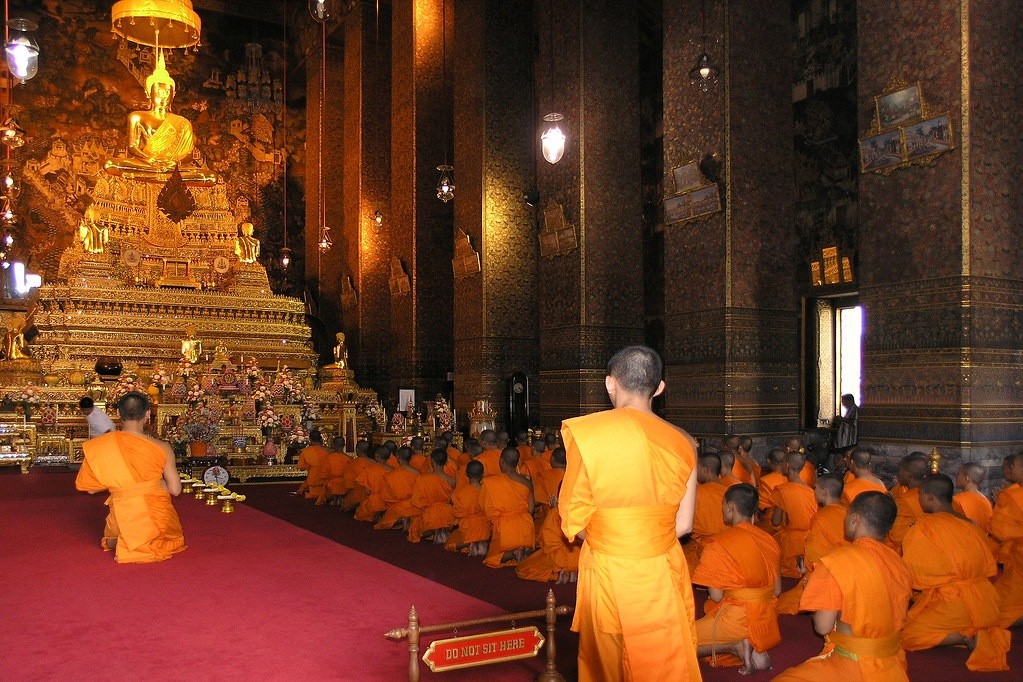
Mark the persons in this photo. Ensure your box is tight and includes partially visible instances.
[104,68,217,186]
[75,392,188,563]
[78,205,109,252]
[78,397,116,437]
[180,328,202,363]
[4,319,30,360]
[323,332,349,368]
[296,347,1023,682]
[234,223,260,263]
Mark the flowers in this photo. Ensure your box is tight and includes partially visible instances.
[179,474,246,502]
[12,355,452,446]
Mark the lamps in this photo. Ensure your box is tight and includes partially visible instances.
[540,0,566,165]
[3,13,40,85]
[317,44,333,254]
[369,209,385,233]
[689,0,718,81]
[0,74,27,150]
[277,0,293,273]
[0,199,18,270]
[0,141,22,199]
[436,0,456,204]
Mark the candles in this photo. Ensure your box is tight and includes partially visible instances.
[454,409,456,423]
[433,417,435,431]
[404,417,407,431]
[482,401,484,411]
[384,408,385,422]
[163,343,248,423]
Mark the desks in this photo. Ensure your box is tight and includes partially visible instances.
[1,385,436,483]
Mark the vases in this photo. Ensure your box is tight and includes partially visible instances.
[264,438,277,455]
[271,383,285,396]
[41,403,56,422]
[190,441,207,457]
[172,383,186,395]
[281,416,292,427]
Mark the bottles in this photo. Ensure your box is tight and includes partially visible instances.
[43,371,60,387]
[70,363,85,384]
